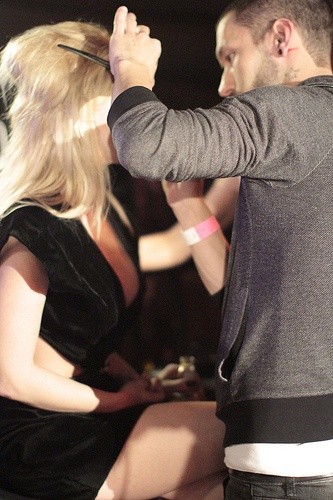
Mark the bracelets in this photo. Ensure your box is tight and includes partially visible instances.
[181,212,221,246]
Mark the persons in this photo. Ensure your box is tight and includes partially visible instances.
[138,170,242,270]
[105,0,332,499]
[1,21,229,500]
